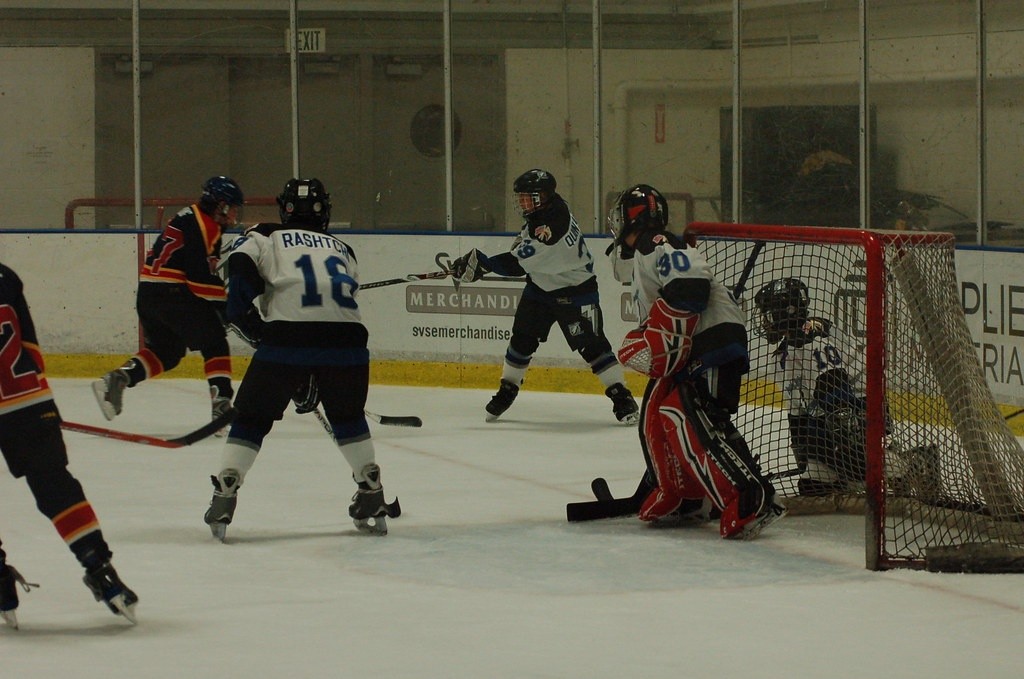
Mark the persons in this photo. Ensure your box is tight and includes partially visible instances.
[204,177,388,520]
[0,263,138,615]
[451,169,641,427]
[92,176,244,438]
[605,184,791,541]
[751,277,942,505]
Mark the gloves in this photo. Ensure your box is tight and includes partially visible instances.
[450,247,492,282]
[292,371,322,414]
[825,407,861,451]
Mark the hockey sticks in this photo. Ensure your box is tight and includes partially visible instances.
[362,409,423,428]
[359,270,455,290]
[313,408,403,519]
[58,405,240,449]
[590,467,803,499]
[565,240,766,521]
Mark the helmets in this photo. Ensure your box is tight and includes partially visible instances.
[279,178,330,231]
[511,168,557,214]
[202,176,245,229]
[622,183,669,228]
[750,276,809,341]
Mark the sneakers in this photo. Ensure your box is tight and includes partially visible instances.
[210,384,232,438]
[204,468,240,541]
[81,561,138,624]
[348,464,401,535]
[92,368,134,420]
[906,443,942,504]
[0,544,38,630]
[888,442,912,499]
[483,378,519,422]
[741,503,789,542]
[605,383,641,425]
